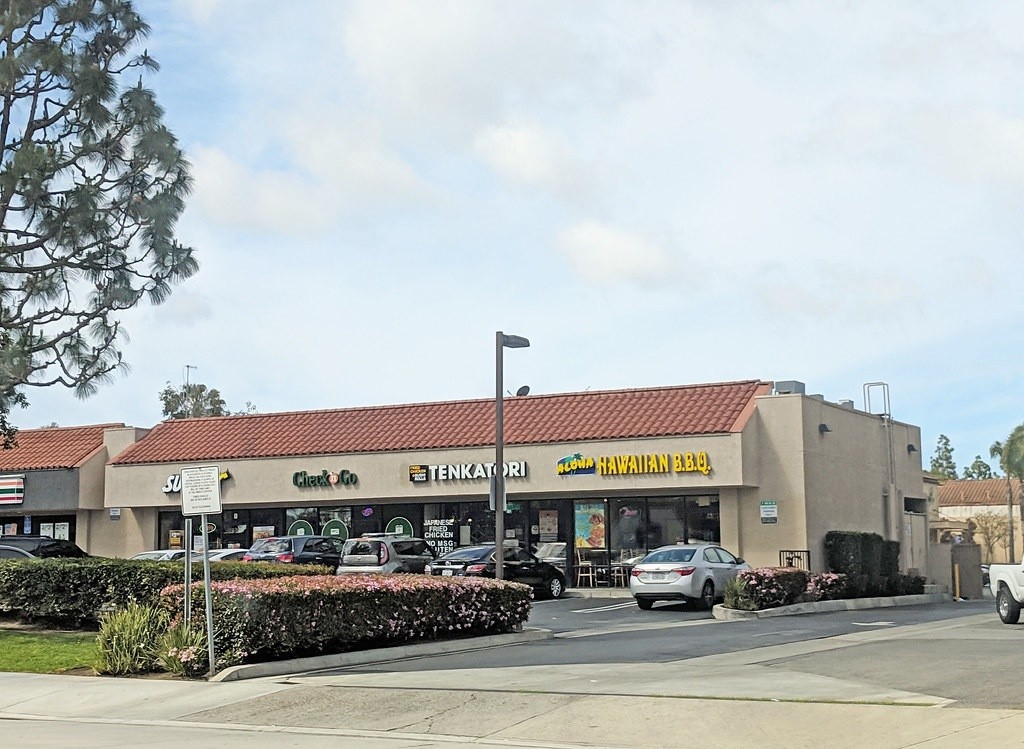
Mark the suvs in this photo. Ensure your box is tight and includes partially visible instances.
[0,535,94,561]
[335,532,440,577]
[242,536,345,575]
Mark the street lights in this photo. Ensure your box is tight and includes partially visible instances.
[186,364,198,420]
[495,331,530,580]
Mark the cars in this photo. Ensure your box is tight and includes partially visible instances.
[425,546,566,600]
[981,563,990,585]
[630,543,753,610]
[129,549,198,563]
[176,549,250,562]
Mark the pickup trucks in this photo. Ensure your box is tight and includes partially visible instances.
[989,554,1024,624]
